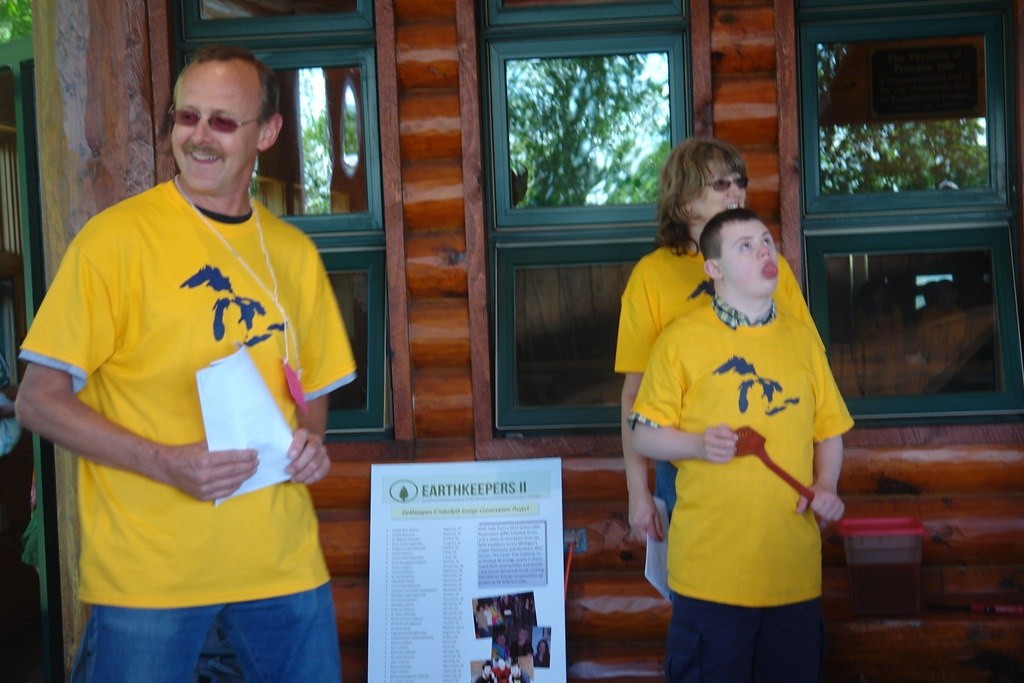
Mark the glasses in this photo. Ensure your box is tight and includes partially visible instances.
[168,103,261,133]
[705,177,748,192]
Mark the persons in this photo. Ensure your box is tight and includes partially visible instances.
[0,42,361,683]
[614,137,828,549]
[475,596,550,683]
[627,210,856,683]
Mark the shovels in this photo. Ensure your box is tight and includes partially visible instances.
[736,426,815,502]
